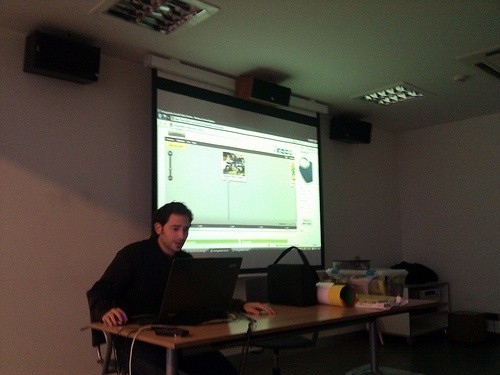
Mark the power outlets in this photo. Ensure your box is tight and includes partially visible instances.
[487,320,500,333]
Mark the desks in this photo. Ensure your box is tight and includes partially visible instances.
[80,302,438,375]
[377,281,449,344]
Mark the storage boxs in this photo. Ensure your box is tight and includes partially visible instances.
[233,277,268,303]
[333,259,371,271]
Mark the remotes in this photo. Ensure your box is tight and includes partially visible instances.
[155,328,189,337]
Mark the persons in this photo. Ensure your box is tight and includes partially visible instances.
[86,202,276,375]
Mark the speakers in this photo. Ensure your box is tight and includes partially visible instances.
[234,76,291,107]
[329,116,372,144]
[23,30,101,85]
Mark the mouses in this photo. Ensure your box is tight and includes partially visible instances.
[114,317,124,327]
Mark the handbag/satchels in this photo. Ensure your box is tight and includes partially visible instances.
[267,247,320,306]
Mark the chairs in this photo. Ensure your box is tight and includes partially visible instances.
[87,289,122,375]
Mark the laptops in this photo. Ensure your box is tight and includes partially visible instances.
[132,257,242,325]
